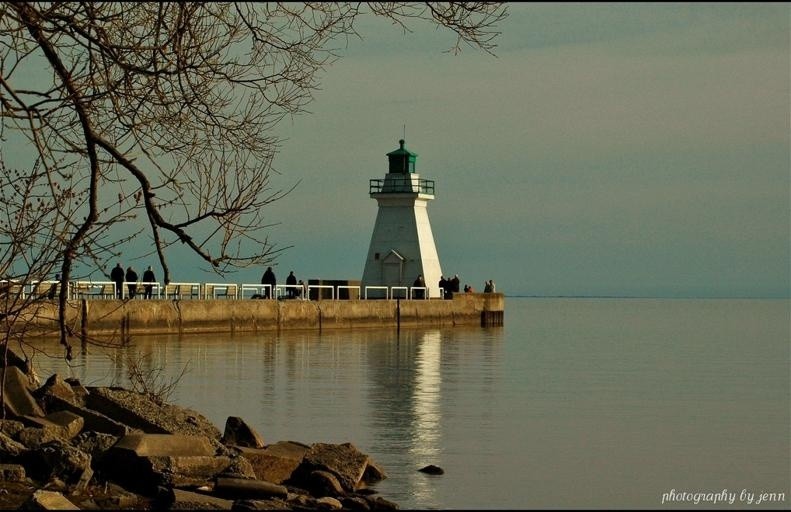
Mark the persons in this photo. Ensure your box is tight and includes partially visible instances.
[299,279,306,298]
[142,265,156,299]
[285,271,296,299]
[438,273,495,300]
[110,263,124,298]
[48,273,60,299]
[261,266,276,299]
[413,274,425,299]
[125,267,138,299]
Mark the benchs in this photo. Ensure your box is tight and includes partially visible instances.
[10,284,240,299]
